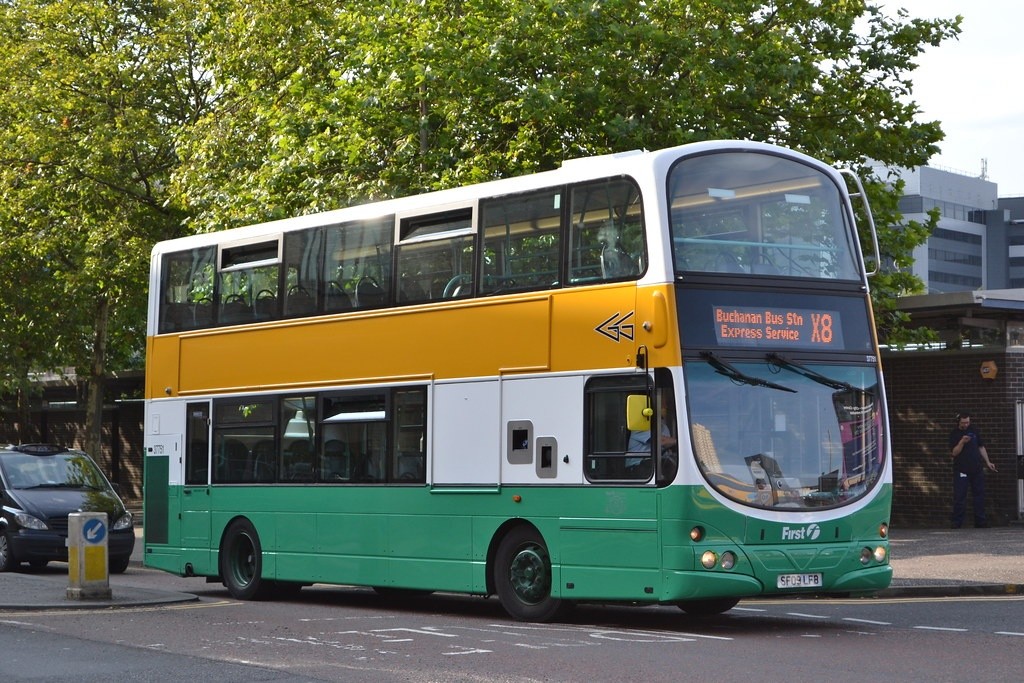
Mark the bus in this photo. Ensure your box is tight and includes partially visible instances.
[142,140,893,623]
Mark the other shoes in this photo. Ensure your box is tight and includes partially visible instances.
[975,521,991,528]
[951,522,961,529]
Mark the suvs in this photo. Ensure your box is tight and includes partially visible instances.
[0,442,136,574]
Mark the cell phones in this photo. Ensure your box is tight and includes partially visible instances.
[967,433,974,437]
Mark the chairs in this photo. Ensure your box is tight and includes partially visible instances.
[188,440,425,487]
[165,242,636,336]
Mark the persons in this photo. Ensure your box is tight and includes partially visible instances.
[624,394,677,479]
[948,412,996,528]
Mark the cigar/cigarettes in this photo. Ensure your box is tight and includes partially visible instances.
[995,470,998,472]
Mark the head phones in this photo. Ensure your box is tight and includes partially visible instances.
[956,412,974,423]
